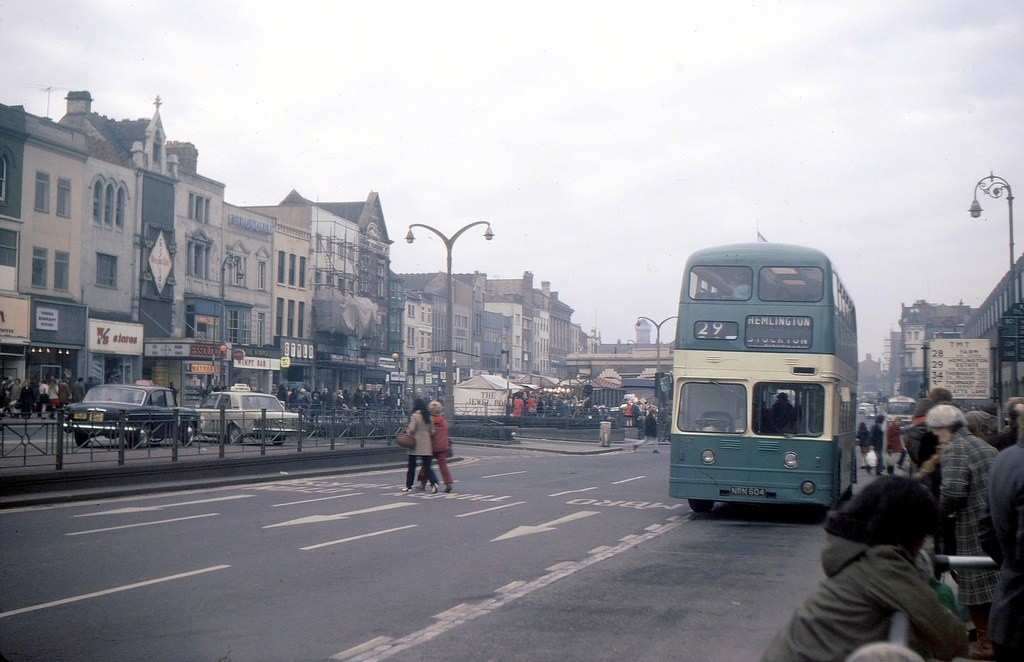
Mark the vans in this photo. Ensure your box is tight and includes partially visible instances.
[887,395,916,433]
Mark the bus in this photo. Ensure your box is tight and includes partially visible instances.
[668,242,858,514]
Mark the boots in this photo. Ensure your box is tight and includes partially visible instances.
[960,601,995,661]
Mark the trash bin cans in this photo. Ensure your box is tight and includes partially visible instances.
[599,421,612,447]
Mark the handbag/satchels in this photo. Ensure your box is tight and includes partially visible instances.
[446,438,453,460]
[866,450,878,467]
[886,451,902,470]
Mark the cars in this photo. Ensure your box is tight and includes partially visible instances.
[858,402,880,419]
[63,385,199,448]
[194,392,299,445]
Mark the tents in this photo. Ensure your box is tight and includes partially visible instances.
[453,375,525,416]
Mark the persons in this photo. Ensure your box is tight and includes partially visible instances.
[633,409,672,454]
[757,388,1024,662]
[271,383,443,420]
[725,392,810,436]
[402,399,440,494]
[415,400,454,493]
[168,382,179,407]
[733,276,751,299]
[506,389,608,419]
[202,381,230,401]
[625,396,658,427]
[0,375,86,420]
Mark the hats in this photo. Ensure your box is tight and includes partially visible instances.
[926,405,969,428]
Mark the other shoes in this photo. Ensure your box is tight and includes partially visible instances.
[860,466,866,469]
[444,484,453,493]
[429,483,439,494]
[653,450,659,453]
[403,486,412,493]
[633,445,638,451]
[415,485,424,490]
[876,470,880,475]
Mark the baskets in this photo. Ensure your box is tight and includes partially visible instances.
[397,428,417,450]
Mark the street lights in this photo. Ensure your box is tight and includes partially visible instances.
[404,221,495,428]
[968,171,1018,396]
[219,253,241,390]
[636,316,661,423]
